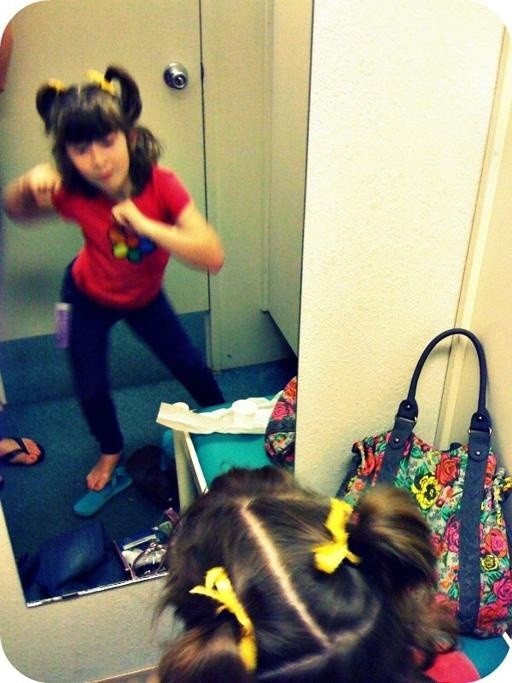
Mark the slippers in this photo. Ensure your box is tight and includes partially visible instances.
[74,466,133,517]
[2,438,45,465]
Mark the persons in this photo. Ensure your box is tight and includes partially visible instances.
[1,435,42,465]
[0,62,228,490]
[163,463,481,683]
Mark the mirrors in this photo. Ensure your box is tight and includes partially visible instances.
[1,1,311,608]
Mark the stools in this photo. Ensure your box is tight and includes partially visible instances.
[164,394,294,516]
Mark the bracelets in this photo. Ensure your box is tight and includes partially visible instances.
[17,175,26,194]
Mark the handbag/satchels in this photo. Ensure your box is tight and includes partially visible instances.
[334,328,512,639]
[264,376,298,475]
